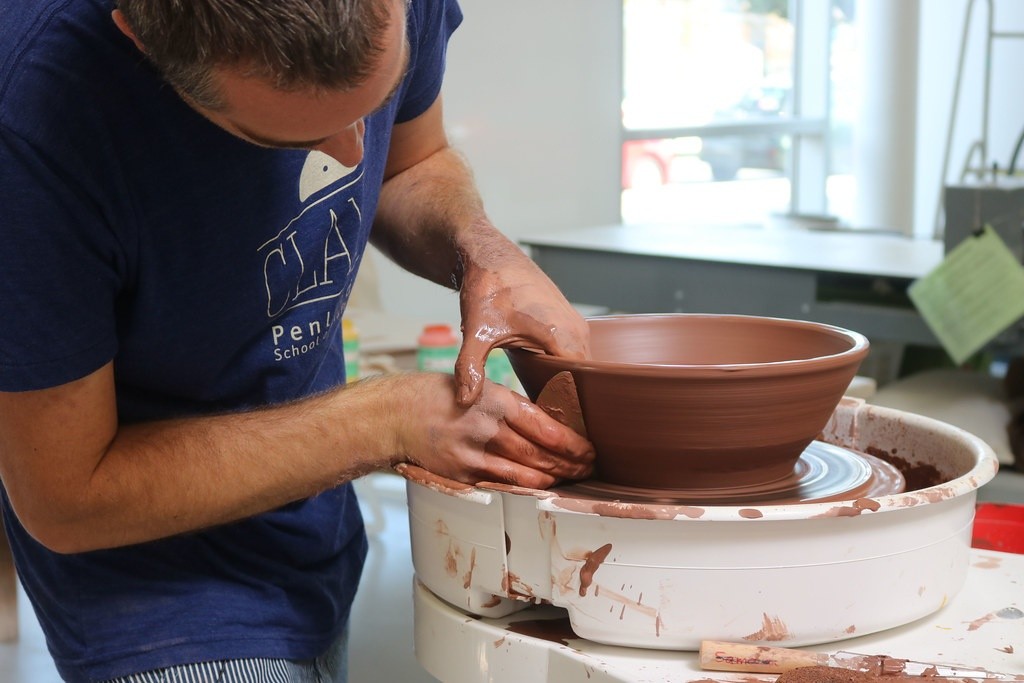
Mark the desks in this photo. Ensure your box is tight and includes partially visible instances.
[520,226,954,385]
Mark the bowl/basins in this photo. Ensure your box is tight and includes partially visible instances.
[504,312,872,494]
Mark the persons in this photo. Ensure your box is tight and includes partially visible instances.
[0,0,597,683]
